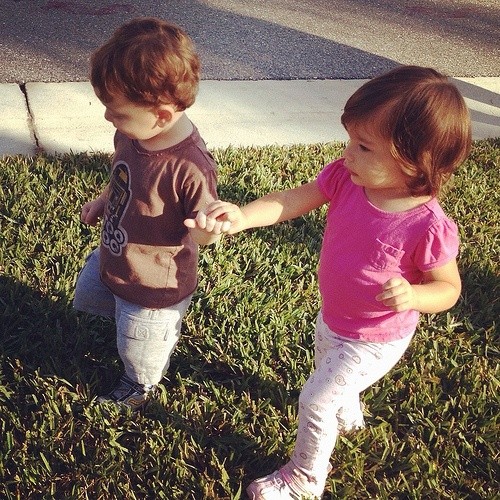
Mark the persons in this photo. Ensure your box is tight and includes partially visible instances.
[205,66,474,500]
[70,19,233,409]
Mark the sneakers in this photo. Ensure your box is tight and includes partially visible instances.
[247,459,329,500]
[97,377,159,418]
